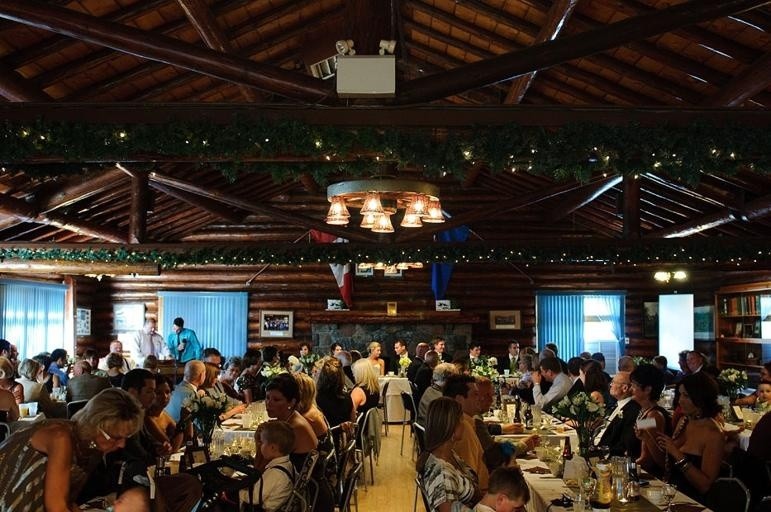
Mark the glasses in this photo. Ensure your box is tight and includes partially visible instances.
[95,427,133,442]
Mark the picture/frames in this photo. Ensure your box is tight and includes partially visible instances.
[382,270,405,280]
[354,262,376,280]
[258,309,294,339]
[488,309,522,330]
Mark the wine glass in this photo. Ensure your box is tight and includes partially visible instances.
[659,388,677,410]
[662,483,679,512]
[742,406,764,432]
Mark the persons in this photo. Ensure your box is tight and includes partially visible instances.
[1,334,771,511]
[129,316,174,370]
[165,315,202,363]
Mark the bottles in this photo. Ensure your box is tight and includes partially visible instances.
[562,436,641,512]
[489,386,534,430]
[154,434,210,479]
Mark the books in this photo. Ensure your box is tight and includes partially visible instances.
[722,294,770,317]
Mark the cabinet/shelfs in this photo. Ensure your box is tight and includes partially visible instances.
[711,279,770,376]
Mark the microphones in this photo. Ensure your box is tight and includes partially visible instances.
[182,338,186,352]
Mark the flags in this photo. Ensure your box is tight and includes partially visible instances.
[428,214,470,301]
[309,214,355,308]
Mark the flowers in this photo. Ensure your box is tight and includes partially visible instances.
[181,353,319,442]
[399,356,500,380]
[718,366,747,403]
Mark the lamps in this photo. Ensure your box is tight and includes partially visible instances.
[654,271,686,283]
[356,235,424,274]
[335,54,396,98]
[325,159,446,233]
[379,39,397,55]
[336,38,356,56]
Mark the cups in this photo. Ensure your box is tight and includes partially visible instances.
[27,402,39,417]
[503,369,510,376]
[388,371,395,377]
[531,404,542,429]
[51,385,67,402]
[18,403,29,418]
[241,401,267,429]
[211,428,256,458]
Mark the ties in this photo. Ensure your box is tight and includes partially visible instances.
[178,333,181,362]
[593,402,618,438]
[148,334,154,356]
[511,358,516,374]
